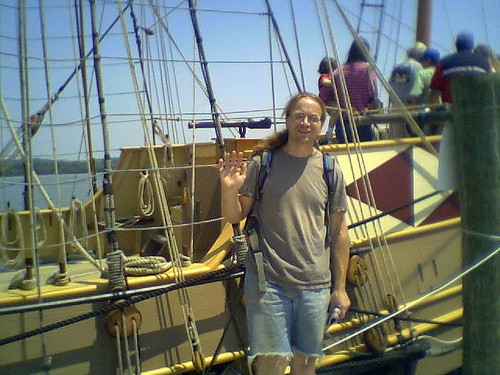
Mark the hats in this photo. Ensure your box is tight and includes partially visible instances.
[406,41,426,56]
[319,57,338,69]
[423,48,440,62]
[454,31,474,49]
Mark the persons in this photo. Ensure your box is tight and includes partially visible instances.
[219,93,350,375]
[318,30,500,145]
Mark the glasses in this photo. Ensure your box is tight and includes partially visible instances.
[288,113,322,124]
